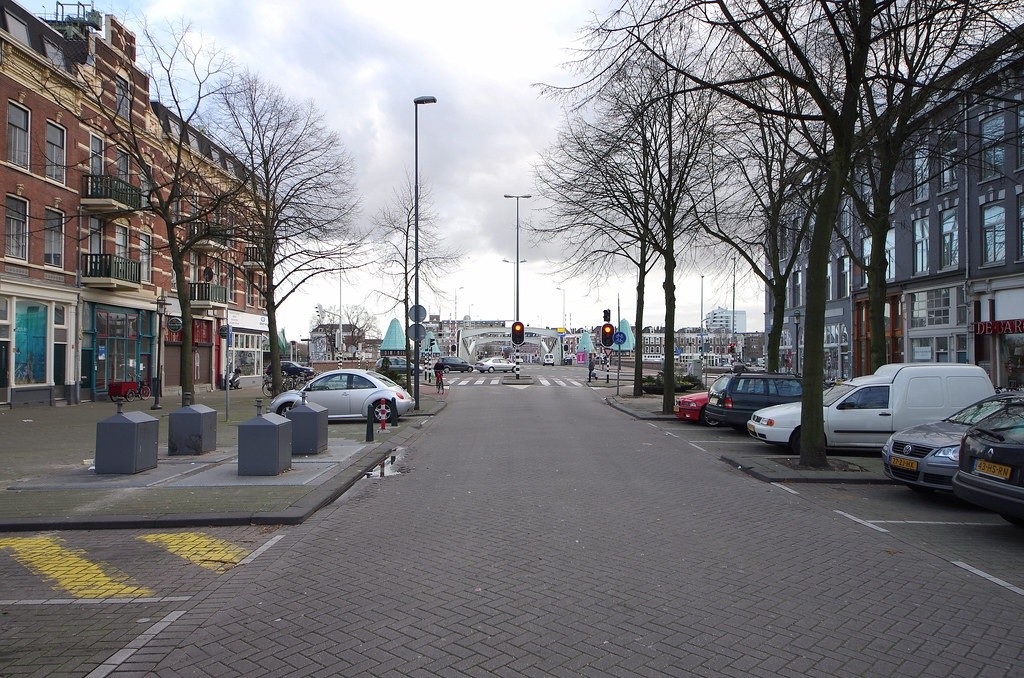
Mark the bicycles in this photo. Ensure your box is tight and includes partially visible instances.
[261,371,308,398]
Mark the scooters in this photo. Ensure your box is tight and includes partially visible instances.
[224,368,241,390]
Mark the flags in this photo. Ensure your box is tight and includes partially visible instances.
[278,328,289,355]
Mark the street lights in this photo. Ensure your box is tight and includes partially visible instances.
[557,287,566,345]
[414,95,438,409]
[454,286,464,357]
[337,243,357,368]
[793,310,801,375]
[701,275,704,348]
[149,295,170,411]
[503,194,532,379]
[731,257,736,342]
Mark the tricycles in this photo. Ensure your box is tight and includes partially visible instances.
[107,374,151,402]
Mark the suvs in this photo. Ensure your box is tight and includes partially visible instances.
[705,373,831,433]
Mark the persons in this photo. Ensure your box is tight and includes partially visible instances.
[235,365,241,377]
[434,358,446,386]
[588,356,598,382]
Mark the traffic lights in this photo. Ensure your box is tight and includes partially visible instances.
[600,324,615,347]
[315,304,325,319]
[730,343,735,353]
[604,309,610,321]
[699,346,702,352]
[511,321,524,346]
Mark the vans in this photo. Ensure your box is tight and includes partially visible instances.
[746,363,996,452]
[543,354,554,365]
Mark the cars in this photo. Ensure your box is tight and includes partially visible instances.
[375,358,424,375]
[674,391,723,428]
[270,369,416,423]
[266,361,314,377]
[474,358,517,373]
[881,390,1024,530]
[433,356,474,373]
